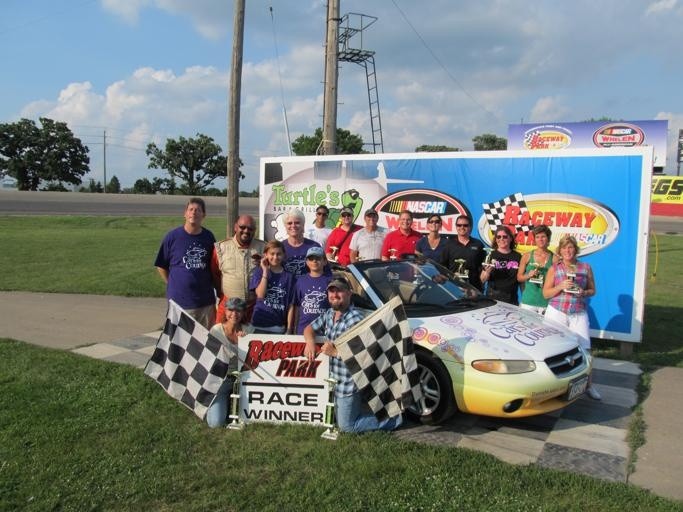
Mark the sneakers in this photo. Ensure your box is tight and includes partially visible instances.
[586,384,601,400]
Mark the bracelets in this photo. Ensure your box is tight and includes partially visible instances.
[261,276,268,279]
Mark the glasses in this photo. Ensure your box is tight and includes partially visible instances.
[342,214,351,217]
[237,223,255,231]
[496,234,510,239]
[316,213,328,216]
[456,224,469,227]
[428,219,441,224]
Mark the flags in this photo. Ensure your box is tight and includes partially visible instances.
[143,298,236,421]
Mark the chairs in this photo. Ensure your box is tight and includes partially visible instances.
[357,268,394,302]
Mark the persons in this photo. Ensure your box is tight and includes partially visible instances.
[205,297,256,430]
[281,208,333,331]
[286,248,332,337]
[304,280,421,432]
[480,226,525,307]
[310,207,487,292]
[517,225,556,315]
[247,240,297,334]
[154,198,216,331]
[210,214,269,324]
[542,236,602,400]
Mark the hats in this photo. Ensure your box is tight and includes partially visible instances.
[339,206,353,215]
[306,246,327,260]
[325,276,351,291]
[224,296,247,311]
[364,209,379,216]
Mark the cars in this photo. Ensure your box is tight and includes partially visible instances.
[332,255,590,427]
